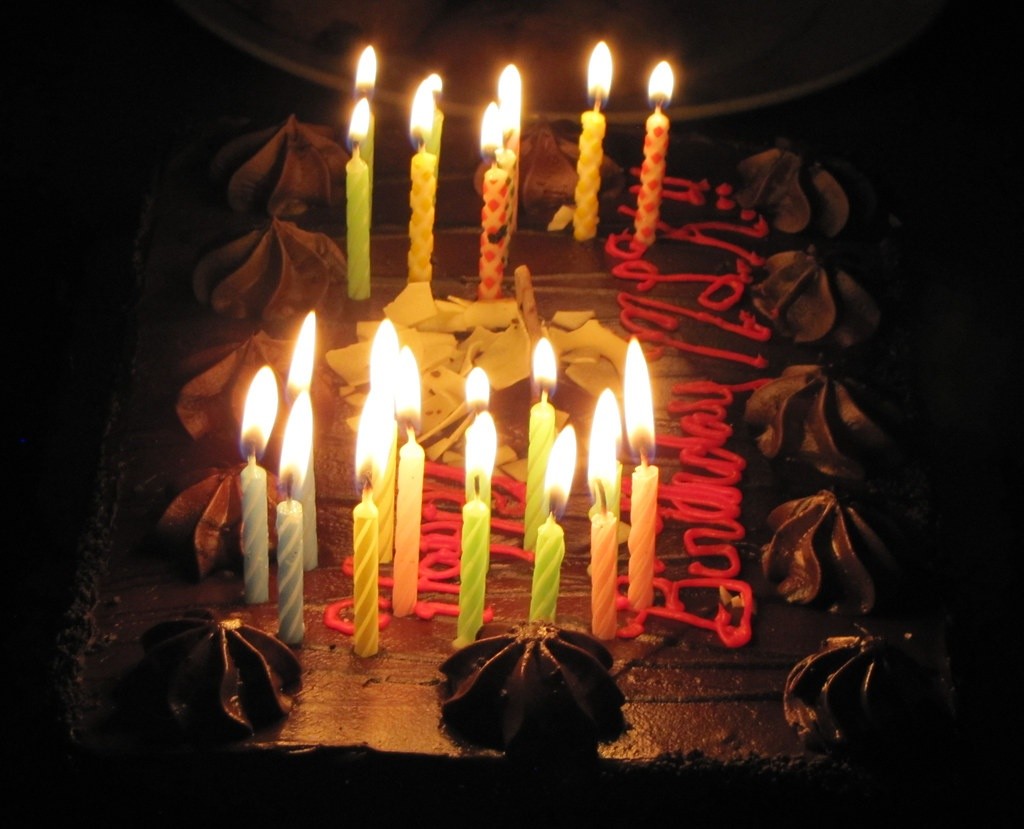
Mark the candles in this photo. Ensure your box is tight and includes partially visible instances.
[347,44,378,301]
[571,41,613,241]
[479,64,522,302]
[634,59,675,246]
[239,311,659,658]
[406,74,444,284]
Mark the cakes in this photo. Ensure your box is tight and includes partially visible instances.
[52,109,906,829]
